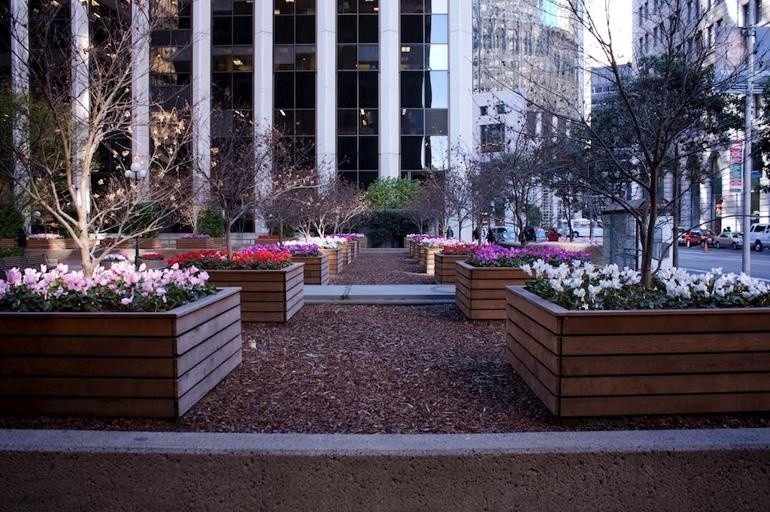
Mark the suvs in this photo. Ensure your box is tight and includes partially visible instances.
[749,223,770,252]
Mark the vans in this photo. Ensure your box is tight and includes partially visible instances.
[492,225,517,243]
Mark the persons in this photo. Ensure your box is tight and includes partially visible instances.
[16,221,27,249]
[447,226,453,239]
[473,228,479,242]
[548,228,559,242]
[487,228,494,243]
[534,225,545,240]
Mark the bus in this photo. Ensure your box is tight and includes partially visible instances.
[555,218,604,239]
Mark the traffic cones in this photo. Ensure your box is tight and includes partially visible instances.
[703,240,709,253]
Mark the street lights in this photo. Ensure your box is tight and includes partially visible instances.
[124,160,148,272]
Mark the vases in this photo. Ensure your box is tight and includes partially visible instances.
[206,262,305,325]
[408,241,434,273]
[292,253,329,287]
[328,238,366,275]
[436,255,544,319]
[6,286,242,419]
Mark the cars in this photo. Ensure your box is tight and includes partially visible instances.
[712,231,744,249]
[678,227,718,248]
[534,227,550,242]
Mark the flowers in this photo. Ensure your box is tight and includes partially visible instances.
[496,283,770,415]
[407,226,475,252]
[264,231,370,253]
[473,238,769,309]
[0,247,296,309]
